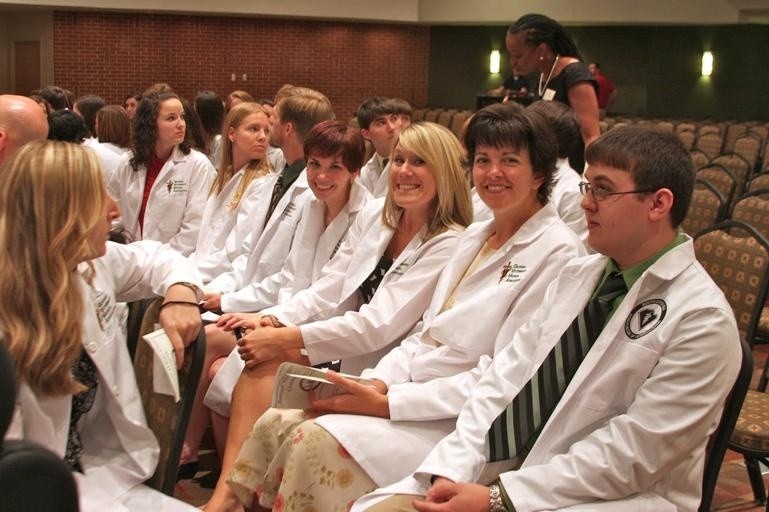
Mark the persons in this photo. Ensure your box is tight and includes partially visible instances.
[505,12,601,180]
[586,61,620,122]
[486,64,529,95]
[1,84,742,510]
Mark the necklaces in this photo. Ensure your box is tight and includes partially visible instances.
[538,51,561,98]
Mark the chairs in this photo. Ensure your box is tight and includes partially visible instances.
[134,300,207,497]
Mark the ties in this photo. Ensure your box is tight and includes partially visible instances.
[484,270,625,462]
[264,175,284,227]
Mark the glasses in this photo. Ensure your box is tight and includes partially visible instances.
[578,181,648,201]
